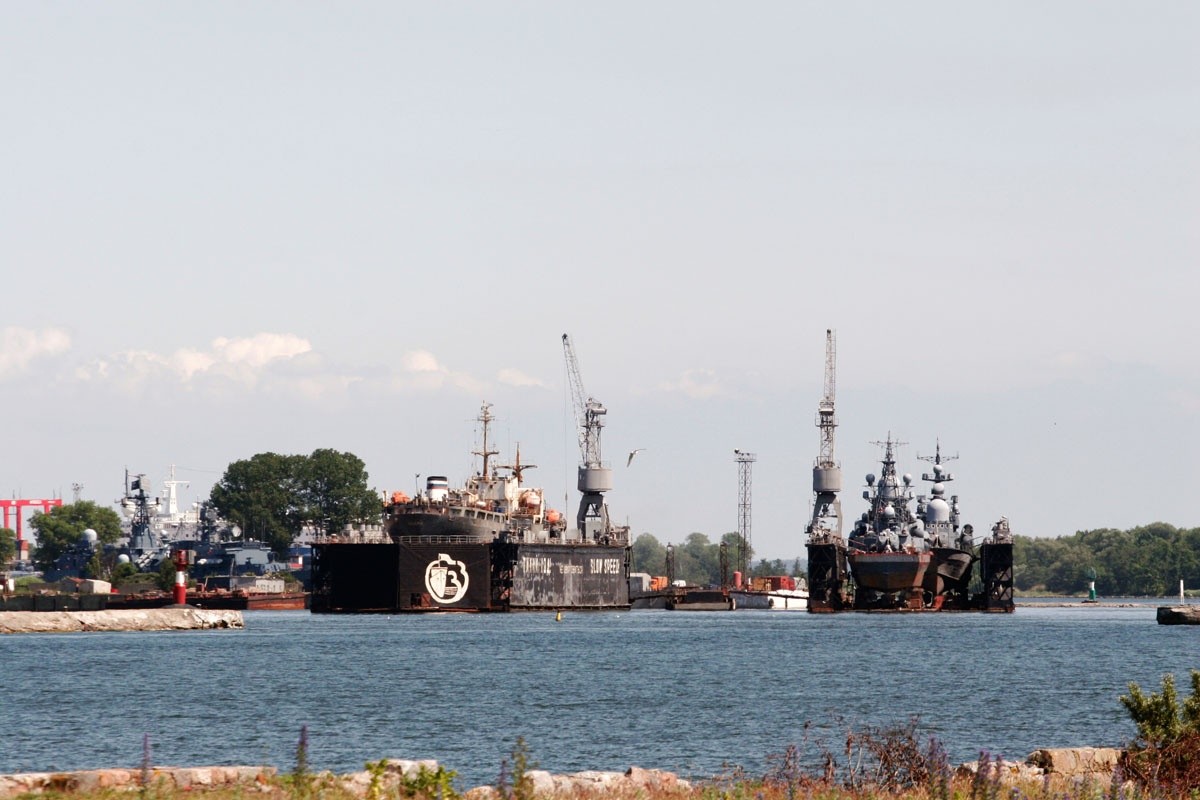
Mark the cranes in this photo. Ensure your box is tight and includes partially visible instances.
[561,333,614,492]
[806,328,842,490]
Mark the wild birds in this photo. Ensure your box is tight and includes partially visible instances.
[627,448,646,468]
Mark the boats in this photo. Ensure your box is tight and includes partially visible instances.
[381,401,569,543]
[845,429,982,613]
